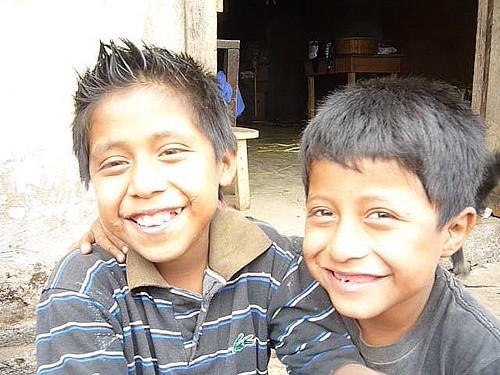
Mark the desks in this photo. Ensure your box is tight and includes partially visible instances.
[303,54,401,124]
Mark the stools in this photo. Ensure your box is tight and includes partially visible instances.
[221,127,259,210]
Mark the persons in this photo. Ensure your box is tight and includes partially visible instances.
[34,38,382,375]
[66,77,500,375]
